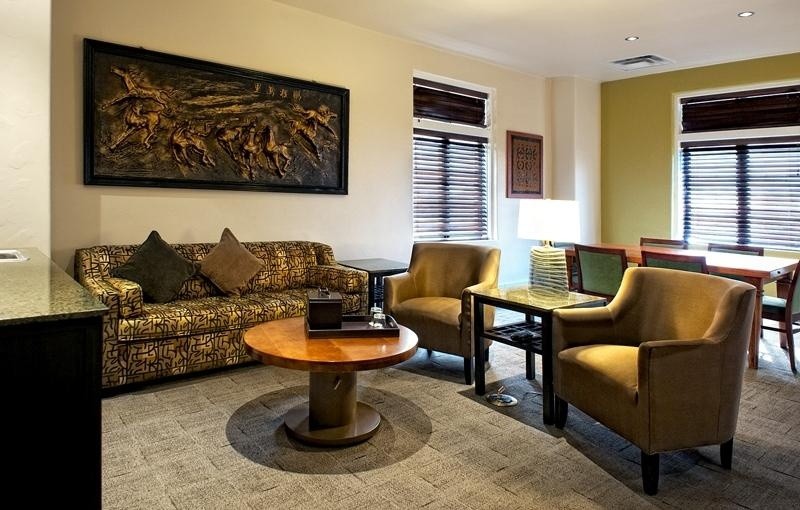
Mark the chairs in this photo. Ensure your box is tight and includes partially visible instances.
[638,236,689,269]
[572,243,628,303]
[755,257,800,377]
[707,241,765,282]
[639,248,710,276]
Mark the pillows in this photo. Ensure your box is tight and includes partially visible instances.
[196,227,267,298]
[110,229,196,306]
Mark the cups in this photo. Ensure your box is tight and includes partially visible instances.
[370,307,384,325]
[373,313,386,328]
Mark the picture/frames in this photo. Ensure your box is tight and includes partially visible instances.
[82,36,350,196]
[506,129,545,199]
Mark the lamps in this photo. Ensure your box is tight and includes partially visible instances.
[516,197,582,298]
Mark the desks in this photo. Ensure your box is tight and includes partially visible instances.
[335,257,411,312]
[470,283,607,428]
[561,243,799,370]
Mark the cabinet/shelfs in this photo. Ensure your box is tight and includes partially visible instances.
[1,245,113,510]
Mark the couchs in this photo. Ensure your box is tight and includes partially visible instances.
[550,266,758,497]
[72,240,371,392]
[383,242,502,387]
